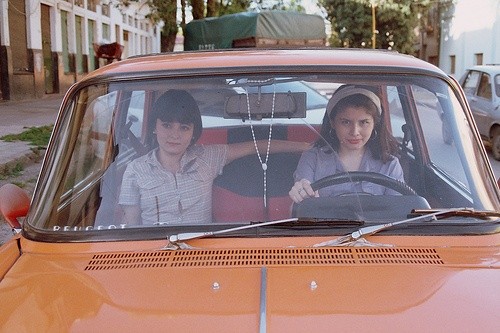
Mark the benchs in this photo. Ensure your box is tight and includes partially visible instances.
[199,123,323,223]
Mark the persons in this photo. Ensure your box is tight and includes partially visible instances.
[288,83,404,203]
[118,90,311,225]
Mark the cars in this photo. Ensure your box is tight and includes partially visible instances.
[0,48,500,333]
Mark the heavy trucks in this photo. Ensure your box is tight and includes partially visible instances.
[182,10,326,52]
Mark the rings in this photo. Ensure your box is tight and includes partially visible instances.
[299,188,304,192]
